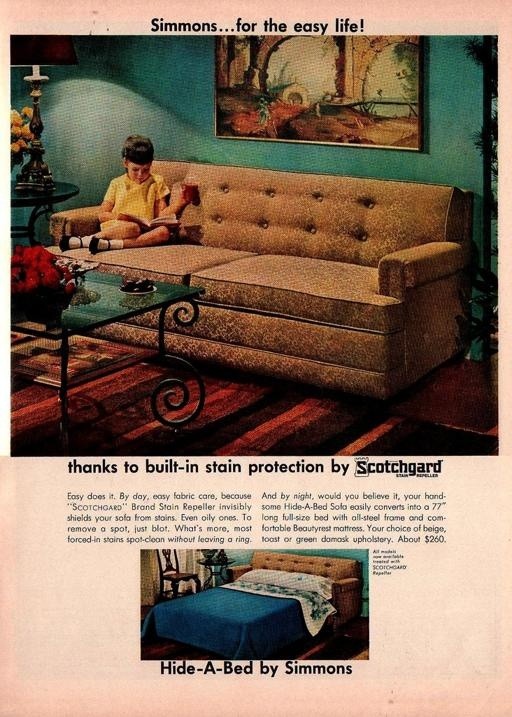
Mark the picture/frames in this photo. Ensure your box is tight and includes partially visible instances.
[212,35,426,152]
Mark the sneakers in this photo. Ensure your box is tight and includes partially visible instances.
[89,236,110,254]
[58,235,83,251]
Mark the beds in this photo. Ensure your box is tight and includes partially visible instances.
[11,180,80,247]
[141,582,337,659]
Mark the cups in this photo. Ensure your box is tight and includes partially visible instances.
[180,175,199,204]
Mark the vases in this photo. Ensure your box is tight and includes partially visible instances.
[17,287,75,321]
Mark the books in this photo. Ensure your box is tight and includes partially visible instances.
[117,212,181,233]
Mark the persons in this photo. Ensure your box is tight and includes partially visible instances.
[56,133,200,254]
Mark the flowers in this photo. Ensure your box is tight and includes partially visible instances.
[10,106,33,168]
[10,244,74,295]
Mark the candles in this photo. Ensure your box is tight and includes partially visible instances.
[33,65,40,77]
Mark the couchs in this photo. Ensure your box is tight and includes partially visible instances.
[225,551,360,632]
[46,158,475,415]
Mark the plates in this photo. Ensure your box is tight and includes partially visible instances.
[118,285,158,296]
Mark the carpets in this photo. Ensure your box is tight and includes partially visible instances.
[11,320,160,390]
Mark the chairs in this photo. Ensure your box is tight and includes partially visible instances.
[156,550,201,604]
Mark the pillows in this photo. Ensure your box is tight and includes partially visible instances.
[234,569,334,600]
